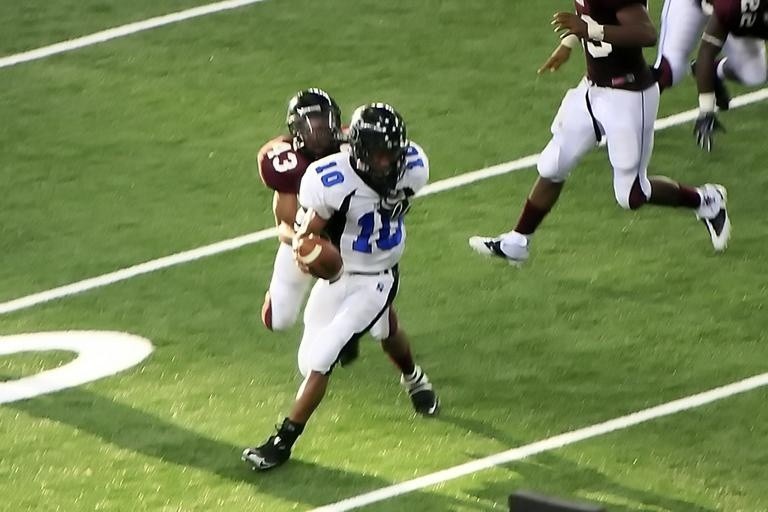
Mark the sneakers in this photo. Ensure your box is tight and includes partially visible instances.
[400,362,441,418]
[695,182,732,251]
[468,229,530,267]
[690,58,731,111]
[241,436,291,471]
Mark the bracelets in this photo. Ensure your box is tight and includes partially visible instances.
[697,93,718,115]
[560,31,579,52]
[587,20,607,43]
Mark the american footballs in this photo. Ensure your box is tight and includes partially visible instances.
[295,232,344,282]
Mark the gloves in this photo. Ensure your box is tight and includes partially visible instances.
[692,110,727,153]
[377,189,413,222]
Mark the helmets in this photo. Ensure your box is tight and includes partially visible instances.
[347,102,407,185]
[285,86,342,154]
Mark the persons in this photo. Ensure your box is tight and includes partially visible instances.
[594,0,768,153]
[259,85,361,367]
[466,2,732,264]
[242,102,443,471]
